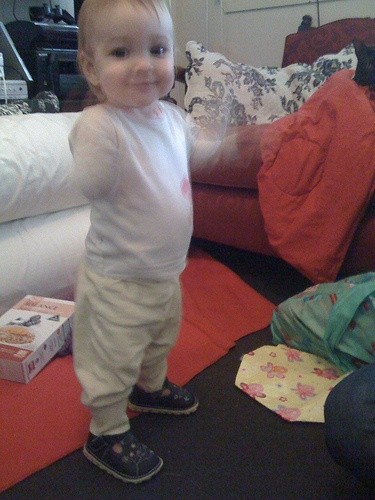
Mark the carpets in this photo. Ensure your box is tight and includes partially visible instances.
[0,242,279,494]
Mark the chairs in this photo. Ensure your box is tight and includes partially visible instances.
[158,15,375,275]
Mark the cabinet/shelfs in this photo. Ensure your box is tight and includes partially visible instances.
[0,20,100,113]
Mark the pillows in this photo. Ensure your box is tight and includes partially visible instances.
[184,39,358,139]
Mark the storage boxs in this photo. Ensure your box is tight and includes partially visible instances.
[0,295,77,384]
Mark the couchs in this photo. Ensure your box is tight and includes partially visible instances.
[0,114,90,314]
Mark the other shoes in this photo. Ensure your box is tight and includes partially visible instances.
[82,431,164,484]
[127,377,200,416]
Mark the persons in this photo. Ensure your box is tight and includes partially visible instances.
[65,0,239,483]
